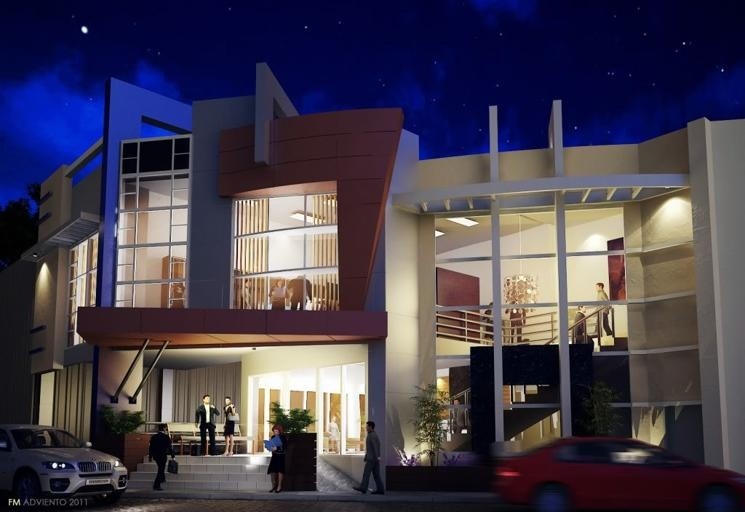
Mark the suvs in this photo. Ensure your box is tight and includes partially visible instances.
[0,422,128,507]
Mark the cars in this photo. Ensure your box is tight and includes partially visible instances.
[492,435,745,511]
